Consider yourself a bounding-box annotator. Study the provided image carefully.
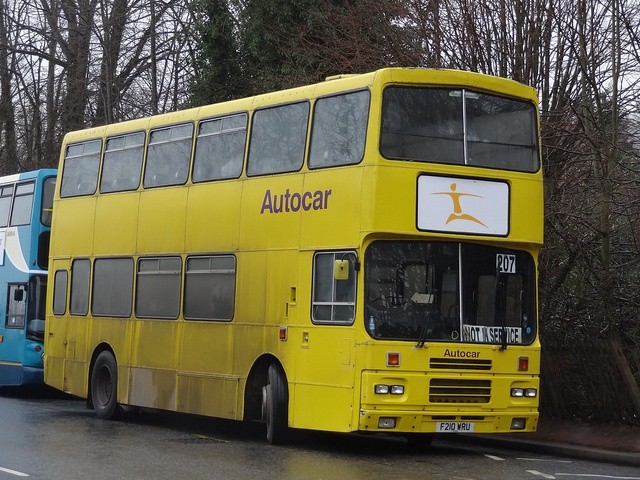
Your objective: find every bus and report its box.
[1,167,58,395]
[37,65,546,448]
